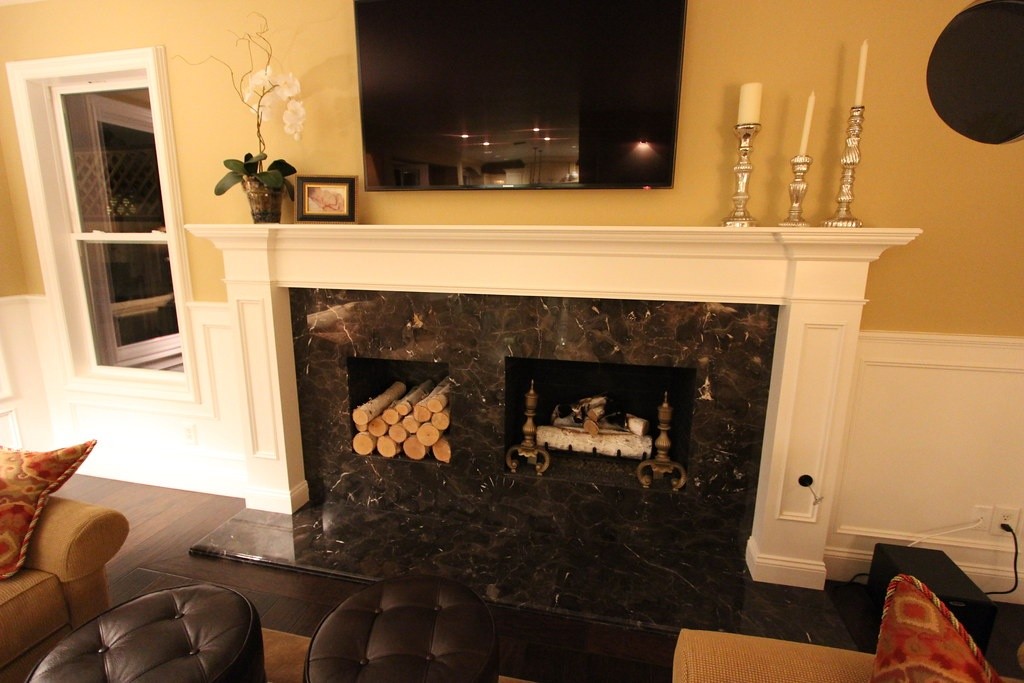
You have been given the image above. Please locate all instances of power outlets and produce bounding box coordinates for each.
[989,505,1019,537]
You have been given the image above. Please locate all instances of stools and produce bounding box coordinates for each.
[300,570,505,683]
[23,575,267,683]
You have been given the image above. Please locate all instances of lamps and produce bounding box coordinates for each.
[632,114,657,142]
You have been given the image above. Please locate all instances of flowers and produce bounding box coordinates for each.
[168,11,308,201]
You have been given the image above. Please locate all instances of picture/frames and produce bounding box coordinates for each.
[295,173,359,225]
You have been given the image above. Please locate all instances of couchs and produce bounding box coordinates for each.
[0,496,136,683]
[672,628,1024,683]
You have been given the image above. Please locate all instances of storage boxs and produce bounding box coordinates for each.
[869,543,999,659]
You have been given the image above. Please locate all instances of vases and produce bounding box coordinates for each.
[242,173,285,221]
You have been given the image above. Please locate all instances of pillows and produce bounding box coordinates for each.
[868,575,1004,683]
[0,438,98,580]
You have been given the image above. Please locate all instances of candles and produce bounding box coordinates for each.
[794,89,818,157]
[732,81,767,126]
[848,39,871,108]
